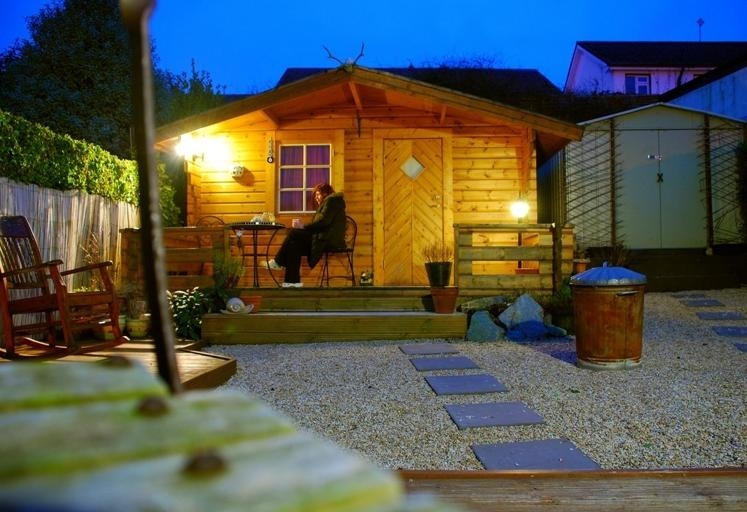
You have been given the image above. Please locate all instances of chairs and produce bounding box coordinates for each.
[1,215,131,362]
[197,216,244,289]
[320,215,357,287]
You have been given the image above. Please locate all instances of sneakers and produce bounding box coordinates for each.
[257,258,284,272]
[278,281,303,288]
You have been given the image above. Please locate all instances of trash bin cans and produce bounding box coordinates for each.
[569,262,647,371]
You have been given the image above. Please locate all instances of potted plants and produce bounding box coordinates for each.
[423,239,455,286]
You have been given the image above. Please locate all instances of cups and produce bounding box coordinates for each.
[292,218,302,229]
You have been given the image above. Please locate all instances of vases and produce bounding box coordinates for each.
[573,259,590,273]
[431,287,458,313]
[240,295,262,313]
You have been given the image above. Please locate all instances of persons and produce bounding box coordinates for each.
[258,182,348,289]
